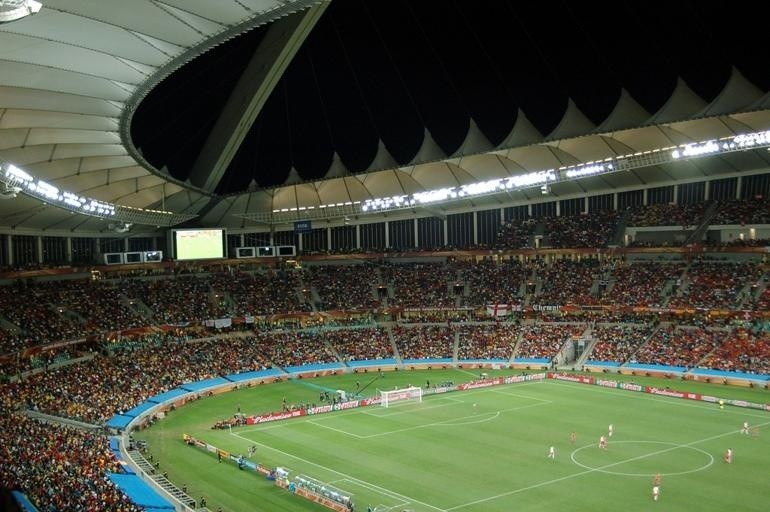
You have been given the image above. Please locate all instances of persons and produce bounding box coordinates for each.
[1,255,339,511]
[571,432,576,442]
[653,472,661,501]
[725,448,731,464]
[609,424,613,438]
[496,197,770,247]
[548,446,555,460]
[319,242,770,407]
[719,399,724,409]
[600,434,605,448]
[741,422,748,435]
